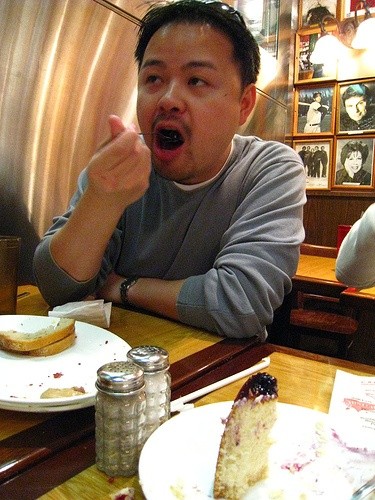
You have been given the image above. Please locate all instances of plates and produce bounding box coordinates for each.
[0,314,133,413]
[137,400,375,500]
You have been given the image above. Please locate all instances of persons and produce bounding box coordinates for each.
[335,202,375,288]
[296,85,372,187]
[33,0,307,341]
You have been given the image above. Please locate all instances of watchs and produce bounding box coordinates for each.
[120,275,141,308]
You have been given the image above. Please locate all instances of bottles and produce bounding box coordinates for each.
[93,344,174,479]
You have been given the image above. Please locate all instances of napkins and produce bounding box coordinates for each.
[48,299,113,329]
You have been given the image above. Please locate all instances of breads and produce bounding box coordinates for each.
[213,371,278,500]
[0,317,76,357]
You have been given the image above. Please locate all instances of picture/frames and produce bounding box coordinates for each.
[340,0,375,22]
[293,25,337,84]
[331,135,375,192]
[336,78,375,134]
[298,0,341,30]
[292,138,334,191]
[292,81,338,136]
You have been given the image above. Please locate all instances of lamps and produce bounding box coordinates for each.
[309,0,375,65]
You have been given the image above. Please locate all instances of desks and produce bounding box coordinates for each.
[290,253,349,310]
[0,277,259,487]
[0,342,375,500]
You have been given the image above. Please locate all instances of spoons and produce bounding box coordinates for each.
[136,131,181,143]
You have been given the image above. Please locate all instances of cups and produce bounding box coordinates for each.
[336,224,352,255]
[0,235,22,315]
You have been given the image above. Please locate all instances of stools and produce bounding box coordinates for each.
[279,307,360,361]
